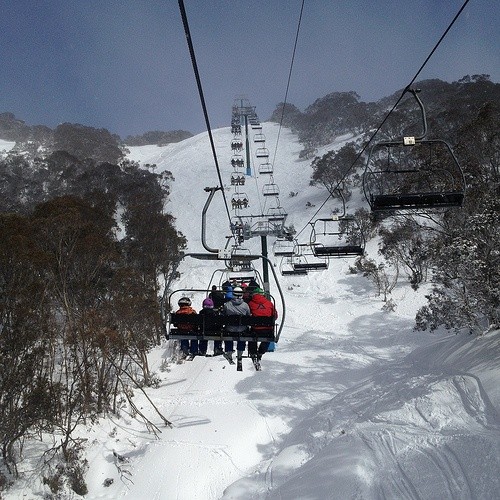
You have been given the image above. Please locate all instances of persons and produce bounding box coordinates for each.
[231,113,241,135]
[231,175,245,185]
[231,198,248,209]
[196,298,225,357]
[173,297,198,356]
[221,287,250,371]
[230,221,249,233]
[207,279,261,309]
[247,284,278,371]
[231,141,243,150]
[231,159,244,167]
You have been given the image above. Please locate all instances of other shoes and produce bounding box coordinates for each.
[255,363,262,371]
[214,348,224,355]
[252,354,258,365]
[238,350,243,357]
[184,350,189,355]
[190,352,197,355]
[199,350,206,355]
[227,352,232,360]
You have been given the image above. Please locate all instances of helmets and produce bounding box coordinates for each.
[252,288,263,295]
[178,297,191,307]
[202,298,214,309]
[233,287,243,299]
